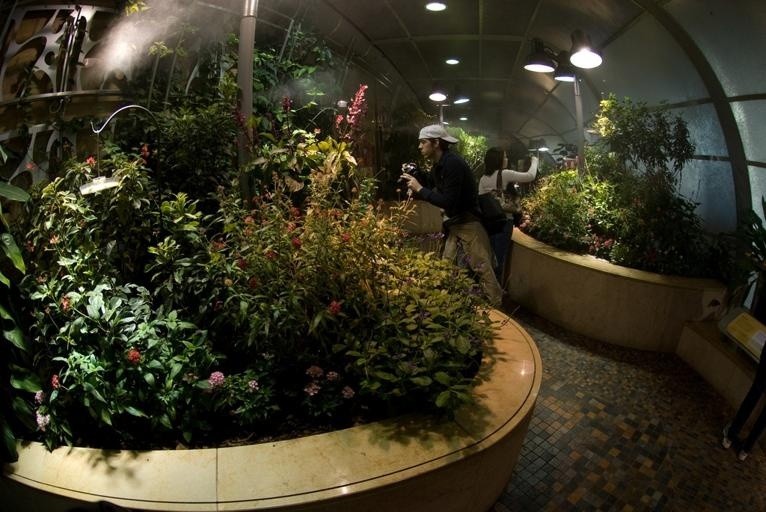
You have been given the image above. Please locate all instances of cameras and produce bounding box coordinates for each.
[397,162,419,188]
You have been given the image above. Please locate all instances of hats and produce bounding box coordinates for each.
[417,124,460,145]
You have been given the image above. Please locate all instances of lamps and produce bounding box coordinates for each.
[520,25,603,83]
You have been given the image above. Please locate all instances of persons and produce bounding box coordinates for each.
[401,124,502,311]
[477,147,539,275]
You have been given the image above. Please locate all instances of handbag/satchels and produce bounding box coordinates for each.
[476,168,523,226]
